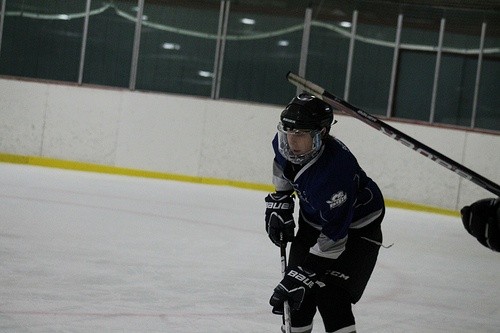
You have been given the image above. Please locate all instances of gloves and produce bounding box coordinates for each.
[270,264,327,314]
[264,192,295,246]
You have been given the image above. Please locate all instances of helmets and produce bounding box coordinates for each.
[280,92,333,143]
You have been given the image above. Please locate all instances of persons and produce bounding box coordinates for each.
[264,92,386,333]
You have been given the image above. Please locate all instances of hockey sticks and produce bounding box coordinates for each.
[280,233,292,333]
[287,71,500,197]
